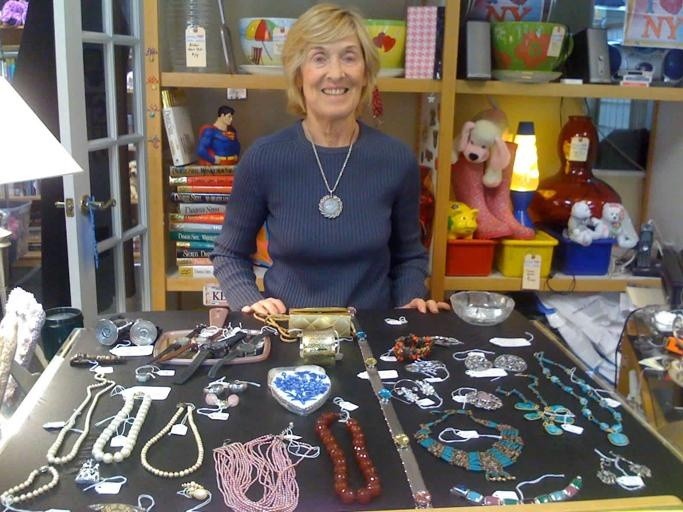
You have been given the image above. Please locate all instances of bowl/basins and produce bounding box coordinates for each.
[363,19,407,66]
[239,19,298,61]
[450,290,516,327]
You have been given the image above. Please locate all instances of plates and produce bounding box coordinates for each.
[240,61,287,78]
[491,66,561,84]
[374,68,405,78]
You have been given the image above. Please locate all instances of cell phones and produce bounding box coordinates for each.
[637,222,655,267]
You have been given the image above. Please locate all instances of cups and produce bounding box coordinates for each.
[493,21,573,69]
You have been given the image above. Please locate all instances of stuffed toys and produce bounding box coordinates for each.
[595,203,639,249]
[450,117,512,189]
[567,201,598,245]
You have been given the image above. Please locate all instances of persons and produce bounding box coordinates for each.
[209,2,451,318]
[196,104,242,165]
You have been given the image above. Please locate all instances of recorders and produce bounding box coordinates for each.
[611,28,683,85]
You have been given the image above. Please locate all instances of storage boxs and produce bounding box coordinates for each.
[550,225,616,275]
[447,239,498,275]
[495,227,559,277]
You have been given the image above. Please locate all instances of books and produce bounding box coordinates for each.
[162,88,195,166]
[168,165,234,279]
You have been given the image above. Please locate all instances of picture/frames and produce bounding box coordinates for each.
[622,0,682,49]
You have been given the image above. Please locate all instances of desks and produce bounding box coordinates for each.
[8,307,683,509]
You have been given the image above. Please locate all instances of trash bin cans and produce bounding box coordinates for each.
[41,306,84,362]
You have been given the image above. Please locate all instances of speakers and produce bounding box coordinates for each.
[466,23,492,79]
[564,30,611,85]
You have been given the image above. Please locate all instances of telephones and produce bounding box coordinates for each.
[631,224,658,277]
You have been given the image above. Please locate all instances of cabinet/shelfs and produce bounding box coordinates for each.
[436,0,682,306]
[141,0,452,307]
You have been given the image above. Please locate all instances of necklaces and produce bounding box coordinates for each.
[592,445,653,491]
[388,332,528,407]
[413,408,524,481]
[1,465,60,506]
[140,402,205,479]
[211,421,320,512]
[449,475,584,506]
[315,411,380,504]
[200,377,248,411]
[497,372,577,436]
[300,117,358,219]
[45,373,115,465]
[91,391,152,465]
[533,351,629,447]
